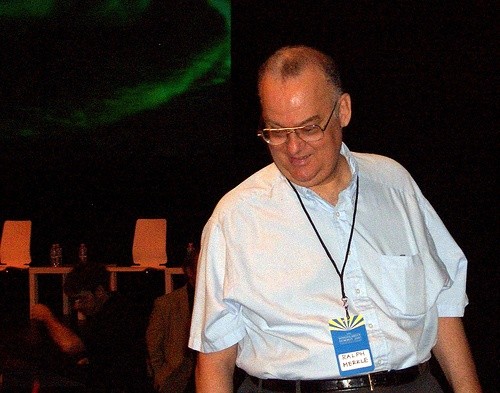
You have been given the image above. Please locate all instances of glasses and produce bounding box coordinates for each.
[257,98,340,144]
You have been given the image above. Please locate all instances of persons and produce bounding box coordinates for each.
[188,46,482,393]
[146,249,200,393]
[31,262,149,393]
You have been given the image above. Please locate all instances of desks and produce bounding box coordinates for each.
[29,267,185,320]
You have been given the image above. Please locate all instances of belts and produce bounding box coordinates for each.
[249,365,422,393]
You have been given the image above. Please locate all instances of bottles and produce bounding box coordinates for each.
[78,243,87,262]
[186,242,195,256]
[50,244,62,268]
